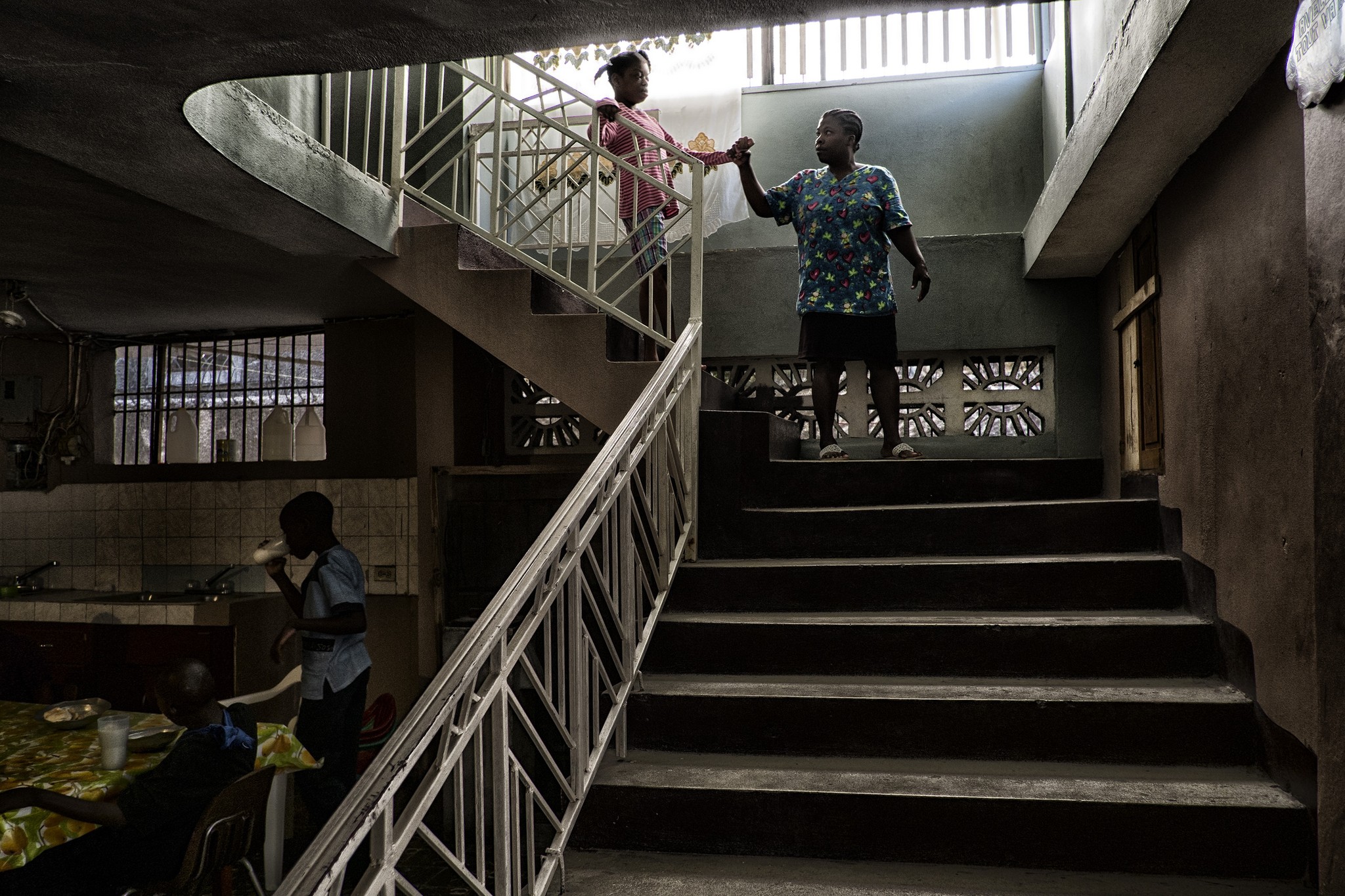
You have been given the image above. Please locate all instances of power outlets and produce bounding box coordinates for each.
[375,567,395,581]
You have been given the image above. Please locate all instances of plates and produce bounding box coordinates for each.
[33,697,111,728]
[127,725,185,751]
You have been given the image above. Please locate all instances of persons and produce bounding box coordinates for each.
[255,491,373,869]
[0,662,256,896]
[588,48,756,367]
[737,107,933,460]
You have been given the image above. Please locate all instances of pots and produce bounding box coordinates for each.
[0,555,60,599]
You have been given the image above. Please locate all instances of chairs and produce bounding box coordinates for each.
[124,763,267,896]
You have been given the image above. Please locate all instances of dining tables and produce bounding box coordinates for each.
[0,699,189,874]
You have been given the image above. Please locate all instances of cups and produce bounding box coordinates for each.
[97,714,128,771]
[252,534,291,565]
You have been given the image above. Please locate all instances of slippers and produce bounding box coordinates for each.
[884,443,926,460]
[818,444,849,460]
